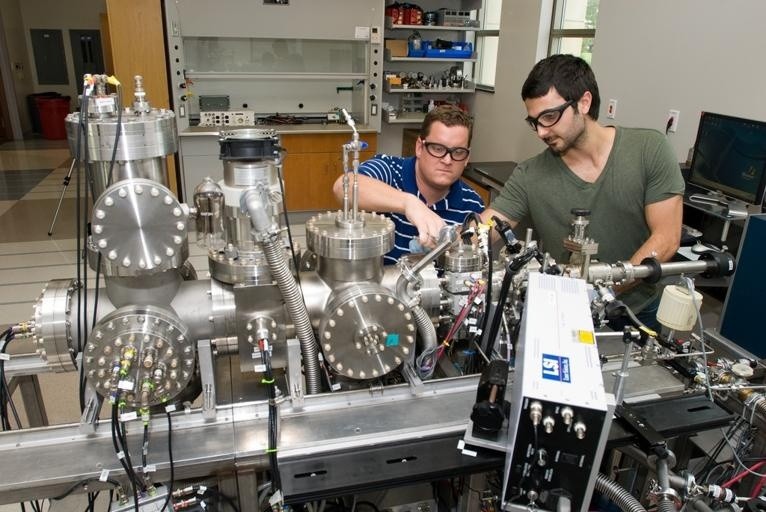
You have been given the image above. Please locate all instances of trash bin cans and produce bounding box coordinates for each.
[27,92,71,140]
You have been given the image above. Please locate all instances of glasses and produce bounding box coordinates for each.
[421,140,471,162]
[525,100,574,131]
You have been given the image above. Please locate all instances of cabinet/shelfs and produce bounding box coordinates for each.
[281,133,377,212]
[384,15,480,125]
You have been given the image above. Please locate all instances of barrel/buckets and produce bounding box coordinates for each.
[34,95,71,139]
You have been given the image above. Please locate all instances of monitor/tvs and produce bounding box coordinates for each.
[688,111,766,217]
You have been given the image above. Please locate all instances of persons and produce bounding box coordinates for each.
[455,55,686,347]
[333,105,486,271]
